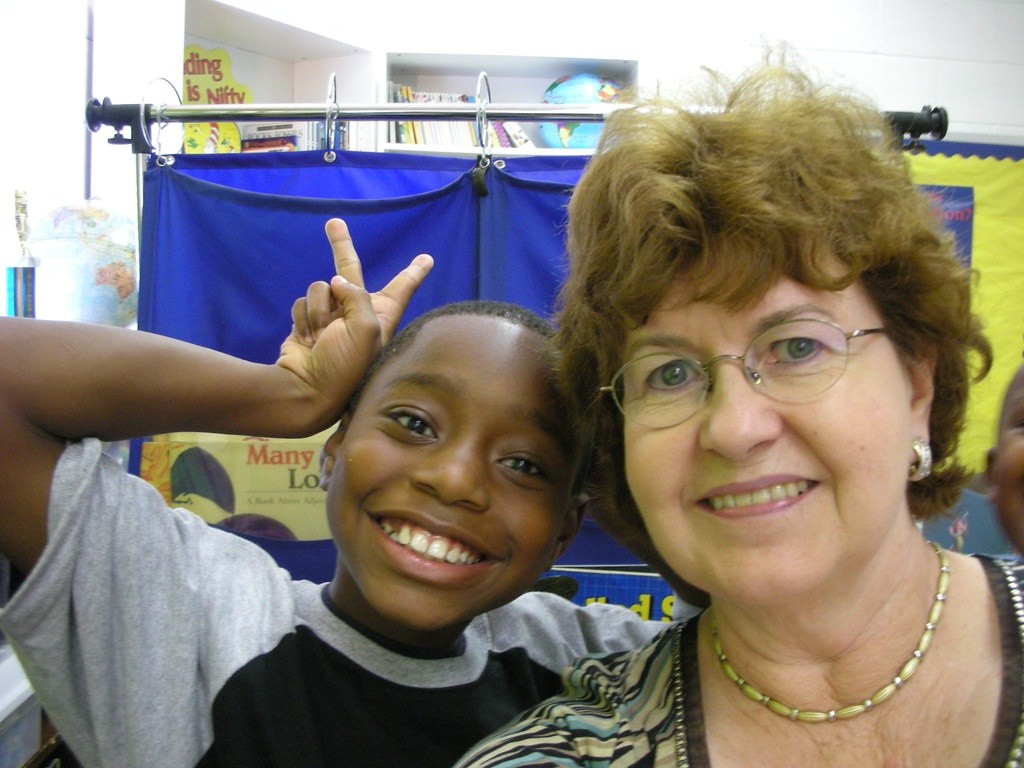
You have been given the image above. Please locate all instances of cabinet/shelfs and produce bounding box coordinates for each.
[0,0,646,218]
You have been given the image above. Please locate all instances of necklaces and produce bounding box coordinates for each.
[710,538,951,723]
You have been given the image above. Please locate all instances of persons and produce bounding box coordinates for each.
[0,218,679,768]
[454,44,1024,768]
[986,366,1024,555]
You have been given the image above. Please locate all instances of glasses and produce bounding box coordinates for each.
[600,318,895,430]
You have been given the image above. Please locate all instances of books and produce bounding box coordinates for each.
[239,80,535,153]
[4,267,36,319]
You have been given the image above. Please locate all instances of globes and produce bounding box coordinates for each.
[540,74,622,149]
